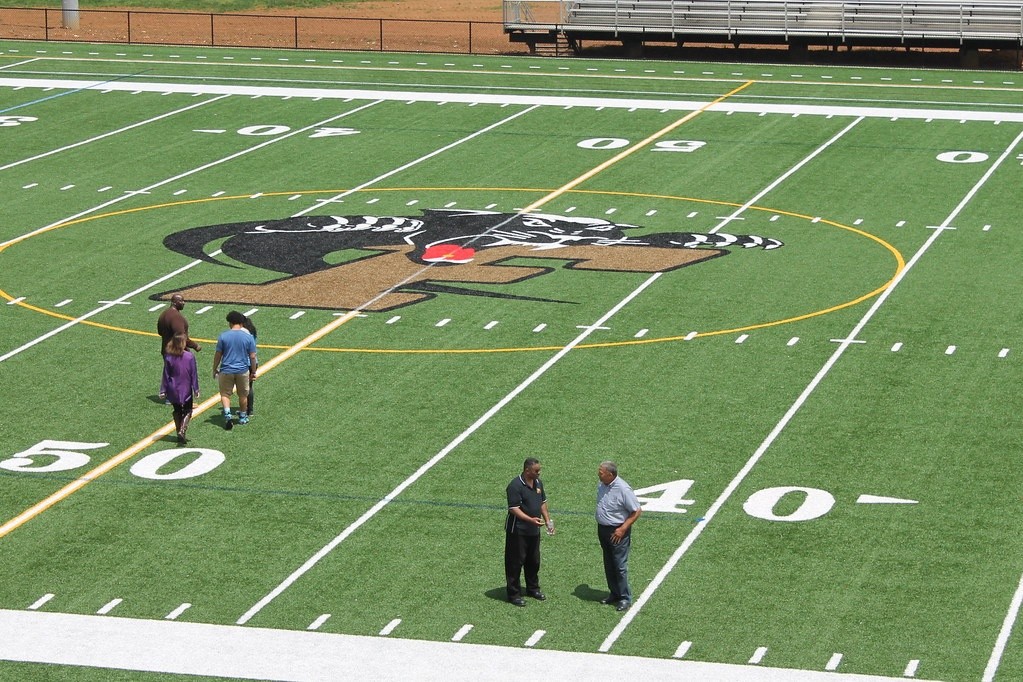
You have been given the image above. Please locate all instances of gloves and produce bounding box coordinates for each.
[159,393,167,400]
[195,392,200,399]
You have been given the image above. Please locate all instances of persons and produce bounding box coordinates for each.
[595,460,640,610]
[504,458,555,606]
[156,295,259,444]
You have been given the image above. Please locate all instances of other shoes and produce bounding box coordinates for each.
[239,411,250,423]
[165,398,172,405]
[225,412,233,428]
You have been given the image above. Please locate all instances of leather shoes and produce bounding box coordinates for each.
[617,599,632,611]
[527,591,546,600]
[509,598,526,606]
[600,596,620,604]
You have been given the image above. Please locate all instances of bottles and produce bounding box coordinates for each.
[215,368,220,378]
[548,520,555,535]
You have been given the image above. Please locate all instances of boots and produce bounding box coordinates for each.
[177,412,190,443]
[173,411,183,442]
